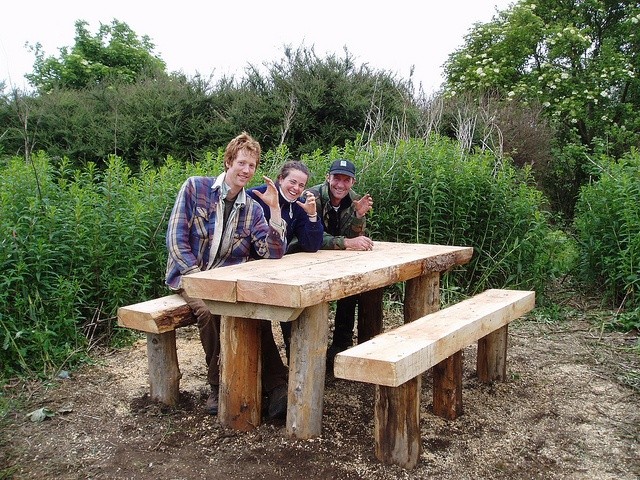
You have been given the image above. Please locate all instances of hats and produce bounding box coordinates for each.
[328,160,357,181]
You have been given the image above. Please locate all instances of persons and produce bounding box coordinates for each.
[302,160,374,368]
[164,129,287,414]
[244,160,323,366]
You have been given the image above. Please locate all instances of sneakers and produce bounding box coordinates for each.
[206,385,220,414]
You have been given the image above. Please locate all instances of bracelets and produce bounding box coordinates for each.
[307,212,319,217]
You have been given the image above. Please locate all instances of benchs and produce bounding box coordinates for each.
[333,287,537,470]
[116,291,198,409]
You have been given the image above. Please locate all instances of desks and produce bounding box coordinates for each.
[182,240,474,441]
